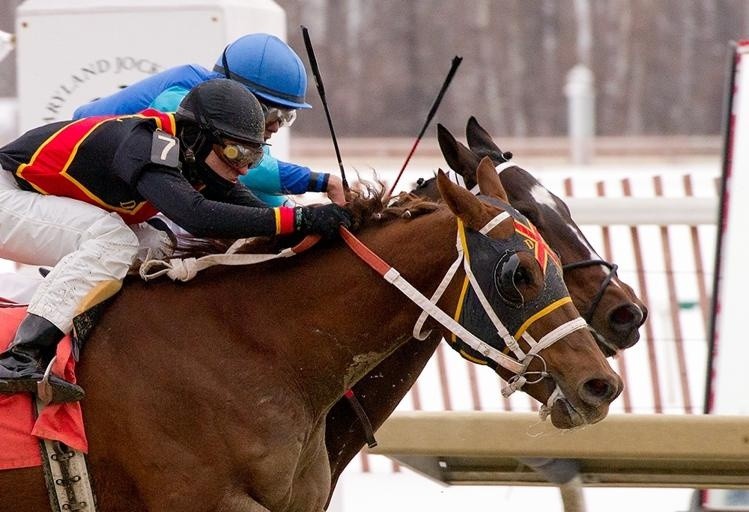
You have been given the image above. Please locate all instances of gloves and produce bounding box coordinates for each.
[294,199,353,237]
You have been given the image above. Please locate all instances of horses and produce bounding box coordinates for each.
[325,115,653,512]
[2,124,623,512]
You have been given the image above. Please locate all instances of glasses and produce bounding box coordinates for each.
[213,138,265,172]
[259,101,298,130]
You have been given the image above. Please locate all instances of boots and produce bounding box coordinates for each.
[0,311,87,405]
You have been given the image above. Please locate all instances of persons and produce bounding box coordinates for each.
[0,78,361,405]
[72,30,359,259]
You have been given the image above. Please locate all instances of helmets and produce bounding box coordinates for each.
[174,76,273,150]
[210,30,315,112]
